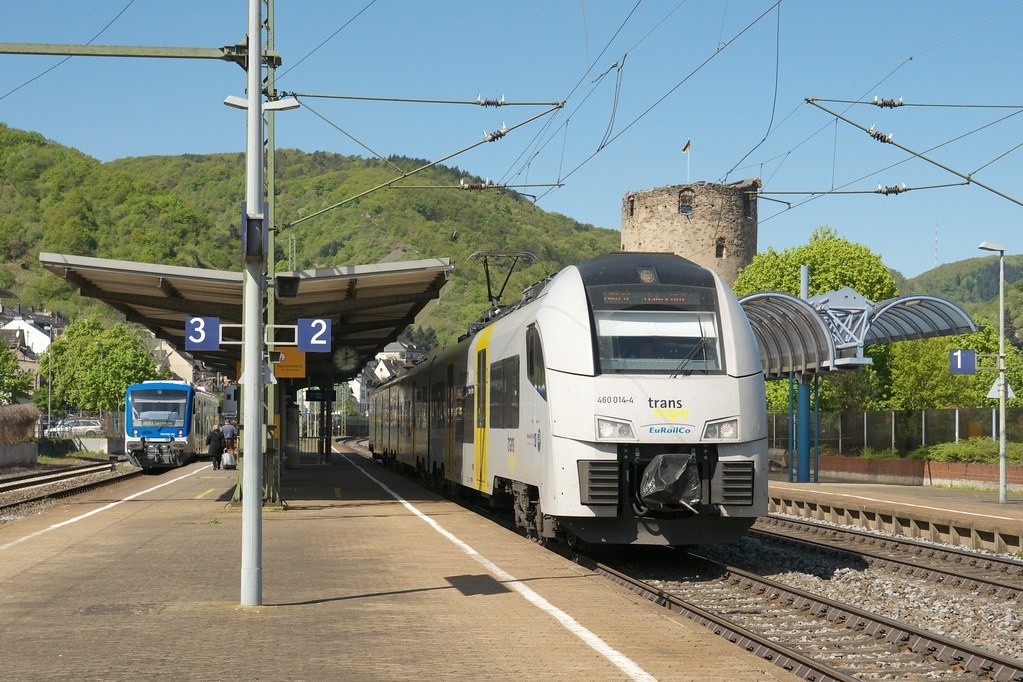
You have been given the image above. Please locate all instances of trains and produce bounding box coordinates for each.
[369,251,769,546]
[125,380,220,469]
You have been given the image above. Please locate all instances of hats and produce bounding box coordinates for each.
[213,425,219,431]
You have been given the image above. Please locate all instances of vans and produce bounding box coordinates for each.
[44,419,104,438]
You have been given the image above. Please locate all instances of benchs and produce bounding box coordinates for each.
[768,448,787,475]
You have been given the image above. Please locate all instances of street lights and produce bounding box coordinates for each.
[223,96,301,606]
[44,325,52,438]
[977,242,1008,504]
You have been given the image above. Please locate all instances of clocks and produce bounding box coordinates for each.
[332,345,361,375]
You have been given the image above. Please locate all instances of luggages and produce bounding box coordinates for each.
[222,449,237,470]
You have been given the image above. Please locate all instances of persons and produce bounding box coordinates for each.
[206,424,227,470]
[220,418,238,454]
[636,338,655,358]
